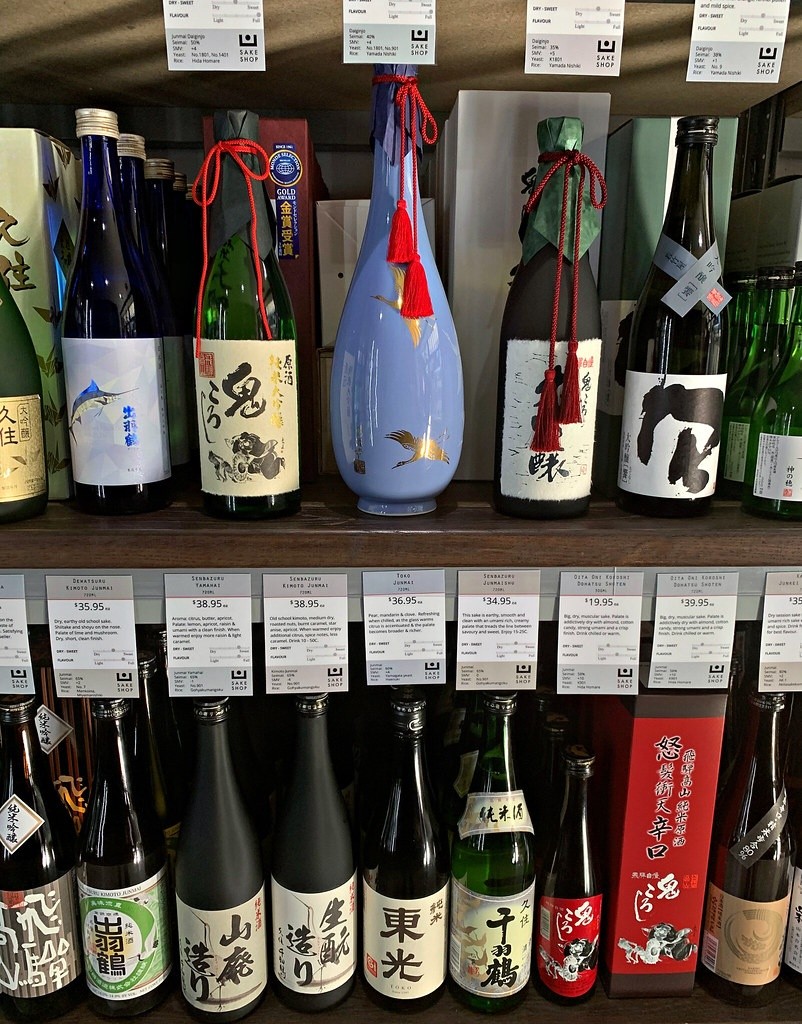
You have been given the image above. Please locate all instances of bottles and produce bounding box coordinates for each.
[2,694,800,1023]
[0,67,802,521]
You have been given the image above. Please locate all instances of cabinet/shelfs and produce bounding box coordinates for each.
[0,0,802,1024]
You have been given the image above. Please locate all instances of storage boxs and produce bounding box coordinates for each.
[588,661,728,997]
[203,90,802,503]
[0,128,86,501]
